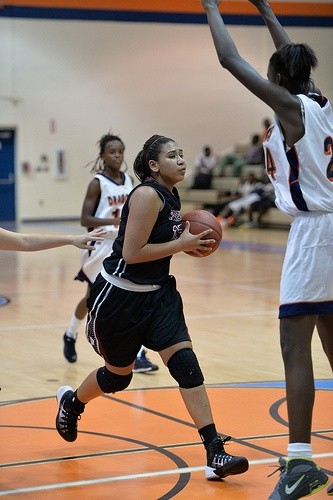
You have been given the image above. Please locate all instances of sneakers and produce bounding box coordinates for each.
[64,334,77,362]
[55,385,81,442]
[204,436,249,479]
[267,456,329,500]
[132,350,159,373]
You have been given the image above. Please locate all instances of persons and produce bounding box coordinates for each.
[64,133,159,373]
[0,226,107,252]
[56,135,249,481]
[200,0,333,500]
[190,119,278,228]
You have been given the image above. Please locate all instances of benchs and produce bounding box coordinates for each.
[173,141,293,226]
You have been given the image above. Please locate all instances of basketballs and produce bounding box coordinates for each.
[180,208,222,258]
